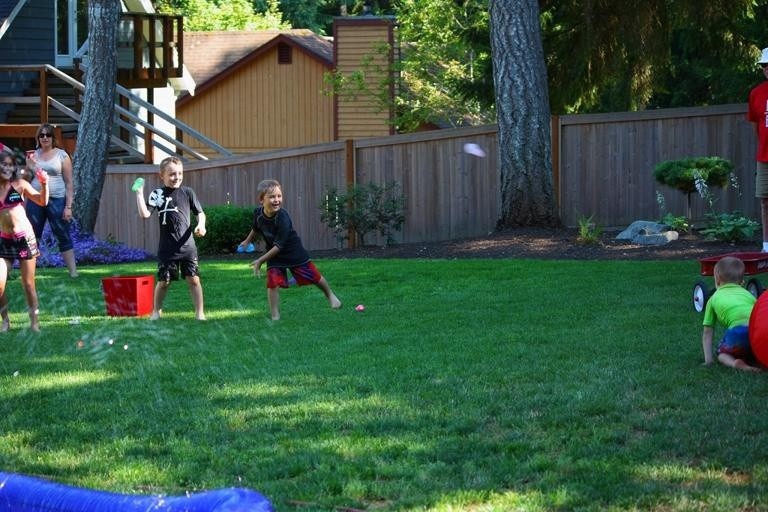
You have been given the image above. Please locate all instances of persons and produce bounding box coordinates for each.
[239,179,342,324]
[702,257,762,373]
[21,123,79,278]
[747,48,768,254]
[0,151,50,333]
[135,157,208,322]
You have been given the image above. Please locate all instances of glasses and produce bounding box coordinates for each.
[37,133,51,139]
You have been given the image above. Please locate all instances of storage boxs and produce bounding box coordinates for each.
[101,275,154,317]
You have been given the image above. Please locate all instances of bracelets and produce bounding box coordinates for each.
[64,205,72,209]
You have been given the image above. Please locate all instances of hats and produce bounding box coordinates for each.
[755,46,767,68]
